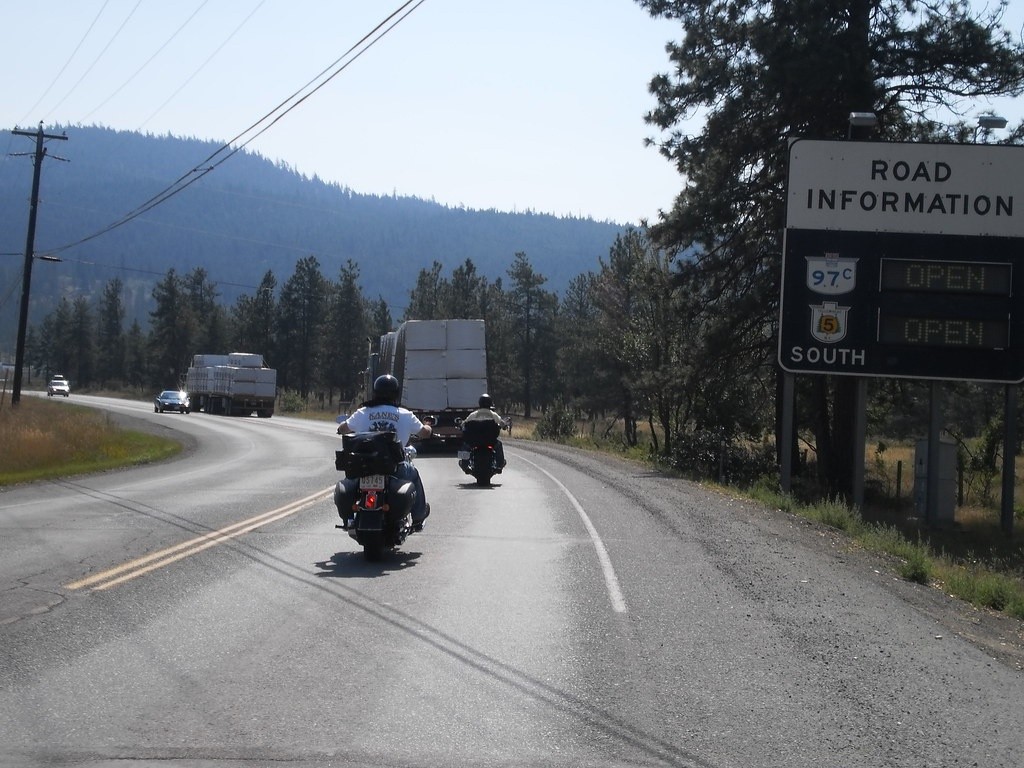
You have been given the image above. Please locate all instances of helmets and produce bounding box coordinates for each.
[479,394,492,408]
[374,375,399,400]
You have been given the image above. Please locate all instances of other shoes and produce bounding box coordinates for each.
[497,460,506,470]
[413,503,430,524]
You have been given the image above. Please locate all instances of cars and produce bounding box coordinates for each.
[155,390,190,414]
[48,380,70,397]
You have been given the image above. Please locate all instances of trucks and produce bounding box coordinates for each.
[179,353,276,418]
[350,320,487,447]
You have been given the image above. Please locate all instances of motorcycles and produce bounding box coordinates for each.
[334,415,444,560]
[454,416,511,485]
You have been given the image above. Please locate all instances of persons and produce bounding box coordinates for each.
[338,375,432,525]
[460,394,509,469]
